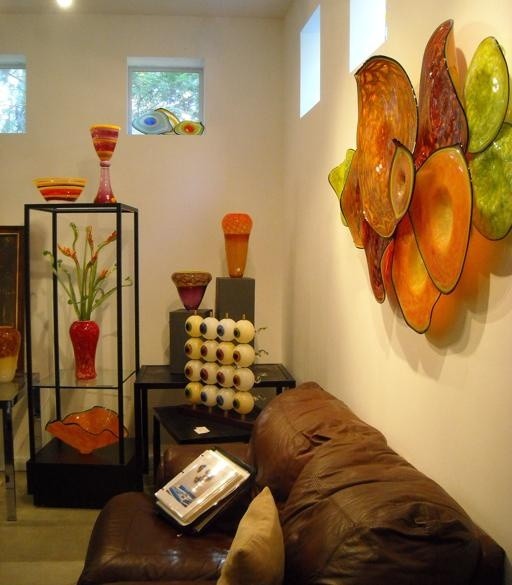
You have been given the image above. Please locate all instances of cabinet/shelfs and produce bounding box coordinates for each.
[25,204,138,509]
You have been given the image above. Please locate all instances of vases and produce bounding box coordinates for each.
[171,273,211,309]
[1,325,21,383]
[223,213,252,278]
[70,322,99,379]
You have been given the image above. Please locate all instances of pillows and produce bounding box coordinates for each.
[214,486,286,584]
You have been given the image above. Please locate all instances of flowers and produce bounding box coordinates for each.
[43,222,133,321]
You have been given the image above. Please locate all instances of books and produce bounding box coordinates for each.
[153,446,257,538]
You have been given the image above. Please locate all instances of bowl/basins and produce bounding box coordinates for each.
[34,177,87,204]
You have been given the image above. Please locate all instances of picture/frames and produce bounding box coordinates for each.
[1,226,24,377]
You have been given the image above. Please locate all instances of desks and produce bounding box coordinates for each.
[1,372,42,520]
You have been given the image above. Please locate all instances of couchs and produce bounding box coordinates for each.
[77,382,506,584]
